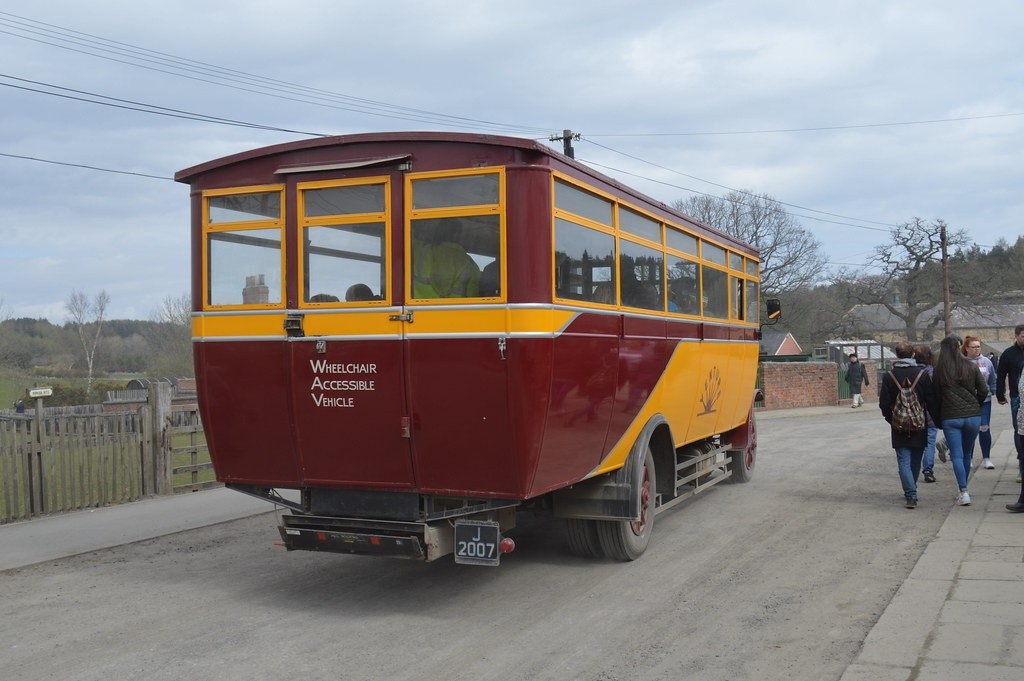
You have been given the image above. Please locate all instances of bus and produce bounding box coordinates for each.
[173,131,784,567]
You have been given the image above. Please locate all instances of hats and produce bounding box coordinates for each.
[850,354,857,357]
[346,284,374,300]
[990,352,994,355]
[948,333,963,345]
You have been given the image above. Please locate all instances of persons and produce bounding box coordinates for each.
[933,338,989,505]
[961,335,996,469]
[912,345,939,483]
[1005,362,1024,513]
[988,352,997,375]
[844,353,869,408]
[309,218,716,319]
[996,325,1024,484]
[934,334,964,463]
[879,342,943,509]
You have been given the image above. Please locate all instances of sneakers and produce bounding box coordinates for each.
[956,491,971,505]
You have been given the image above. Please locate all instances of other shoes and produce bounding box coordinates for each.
[936,441,948,462]
[852,405,855,408]
[1006,502,1024,512]
[925,471,936,482]
[858,401,864,407]
[904,497,918,507]
[983,459,995,469]
[1015,473,1022,483]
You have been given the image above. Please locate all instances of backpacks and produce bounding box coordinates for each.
[887,369,927,438]
